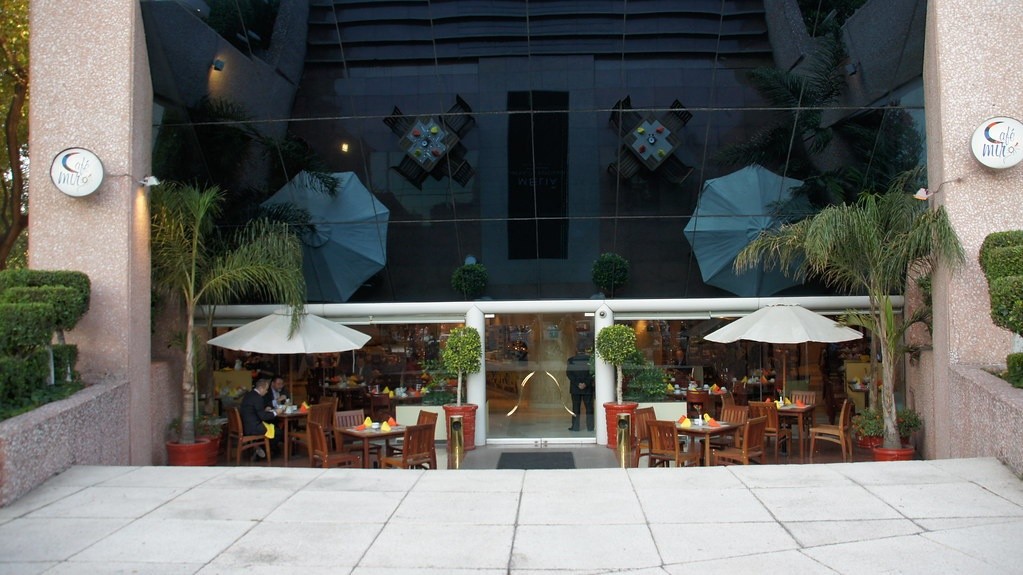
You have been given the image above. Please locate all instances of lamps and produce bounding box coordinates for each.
[139,174,160,188]
[913,187,928,201]
[212,59,225,72]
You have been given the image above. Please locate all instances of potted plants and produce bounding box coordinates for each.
[451,261,490,298]
[715,19,850,181]
[729,192,966,460]
[590,250,634,298]
[148,178,310,467]
[442,325,483,449]
[161,96,315,171]
[595,325,640,450]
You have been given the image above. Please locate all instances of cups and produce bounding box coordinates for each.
[401,392,407,397]
[703,384,708,388]
[372,423,379,428]
[721,387,727,392]
[278,399,297,413]
[694,419,699,425]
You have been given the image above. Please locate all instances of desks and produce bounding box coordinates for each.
[779,402,817,460]
[397,113,460,174]
[333,422,404,468]
[671,420,742,466]
[274,410,307,463]
[622,113,683,171]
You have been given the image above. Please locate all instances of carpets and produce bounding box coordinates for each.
[494,451,577,469]
[505,88,570,260]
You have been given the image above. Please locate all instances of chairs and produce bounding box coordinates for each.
[439,154,466,177]
[383,106,412,136]
[444,94,474,140]
[634,390,854,466]
[609,94,643,132]
[658,154,696,185]
[225,393,438,471]
[389,155,424,184]
[662,98,693,131]
[607,155,643,186]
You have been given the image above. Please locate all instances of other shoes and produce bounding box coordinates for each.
[568,427,579,431]
[255,447,265,458]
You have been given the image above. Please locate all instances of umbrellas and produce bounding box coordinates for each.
[252,170,390,301]
[683,164,822,298]
[205,304,373,407]
[704,305,864,428]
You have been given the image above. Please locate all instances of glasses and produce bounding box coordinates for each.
[275,382,284,386]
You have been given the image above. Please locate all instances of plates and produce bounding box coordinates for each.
[696,388,702,390]
[680,388,688,390]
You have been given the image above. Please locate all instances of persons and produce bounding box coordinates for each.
[314,350,381,411]
[239,373,298,458]
[361,345,394,384]
[733,343,752,378]
[566,342,594,432]
[750,345,764,369]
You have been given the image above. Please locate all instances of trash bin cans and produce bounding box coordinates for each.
[686,390,710,418]
[371,393,396,423]
